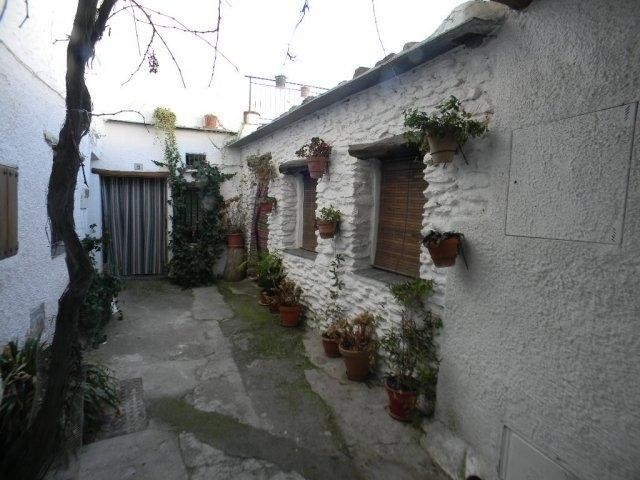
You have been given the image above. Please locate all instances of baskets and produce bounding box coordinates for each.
[318,221,337,239]
[428,237,458,267]
[307,156,325,178]
[261,203,272,213]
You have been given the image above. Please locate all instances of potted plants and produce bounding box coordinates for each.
[246,96,491,185]
[250,193,470,271]
[233,247,443,423]
